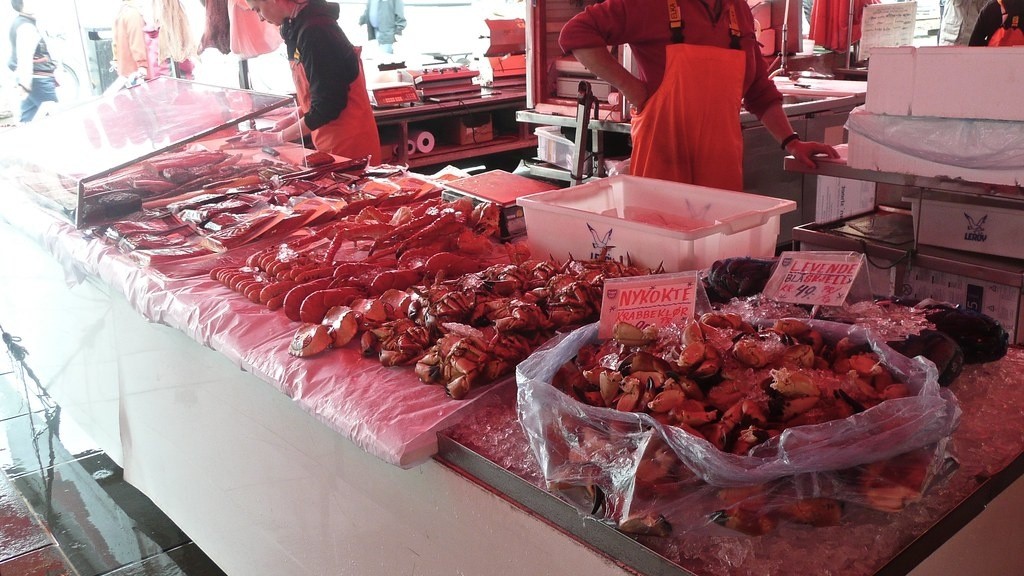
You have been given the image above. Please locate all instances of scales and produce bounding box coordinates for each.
[441,169,562,244]
[366,81,421,108]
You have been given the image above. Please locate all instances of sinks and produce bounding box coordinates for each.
[735,94,827,112]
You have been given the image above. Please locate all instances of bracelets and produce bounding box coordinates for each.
[781,132,801,150]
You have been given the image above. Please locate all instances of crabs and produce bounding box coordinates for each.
[361,246,668,400]
[546,316,962,537]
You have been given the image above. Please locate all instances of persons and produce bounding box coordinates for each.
[558,0,841,193]
[969,0,1024,46]
[240,0,381,167]
[358,0,407,54]
[7,0,60,122]
[114,0,149,77]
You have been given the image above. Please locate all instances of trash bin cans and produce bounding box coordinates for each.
[84,27,118,95]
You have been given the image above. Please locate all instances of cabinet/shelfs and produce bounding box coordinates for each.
[786,146,1024,349]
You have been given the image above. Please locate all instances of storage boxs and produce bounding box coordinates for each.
[449,113,493,145]
[846,43,1024,190]
[800,240,1020,346]
[902,189,1024,260]
[747,0,776,56]
[533,124,575,167]
[516,174,796,275]
